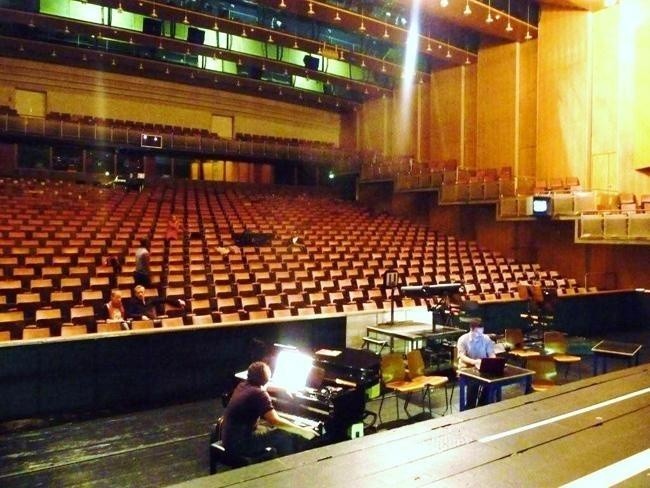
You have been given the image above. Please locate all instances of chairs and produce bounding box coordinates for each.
[379,350,449,421]
[505,329,581,381]
[360,149,649,240]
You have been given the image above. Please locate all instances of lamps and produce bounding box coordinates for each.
[486,0,532,40]
[279,0,472,65]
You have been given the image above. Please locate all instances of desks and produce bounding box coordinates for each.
[456,363,536,411]
[367,321,467,375]
[590,339,642,377]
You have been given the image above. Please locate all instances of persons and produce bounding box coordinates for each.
[457,316,499,410]
[133,234,152,288]
[126,284,185,320]
[100,291,128,323]
[166,214,186,240]
[220,361,322,463]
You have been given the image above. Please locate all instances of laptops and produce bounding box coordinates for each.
[475,358,505,372]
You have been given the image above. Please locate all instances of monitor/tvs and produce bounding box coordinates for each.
[141,134,163,148]
[526,196,552,217]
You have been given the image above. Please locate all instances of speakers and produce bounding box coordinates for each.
[187,28,205,44]
[143,18,161,35]
[304,55,319,70]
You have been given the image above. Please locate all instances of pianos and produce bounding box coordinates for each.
[400,283,462,297]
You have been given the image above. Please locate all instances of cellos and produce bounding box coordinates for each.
[223,342,380,445]
